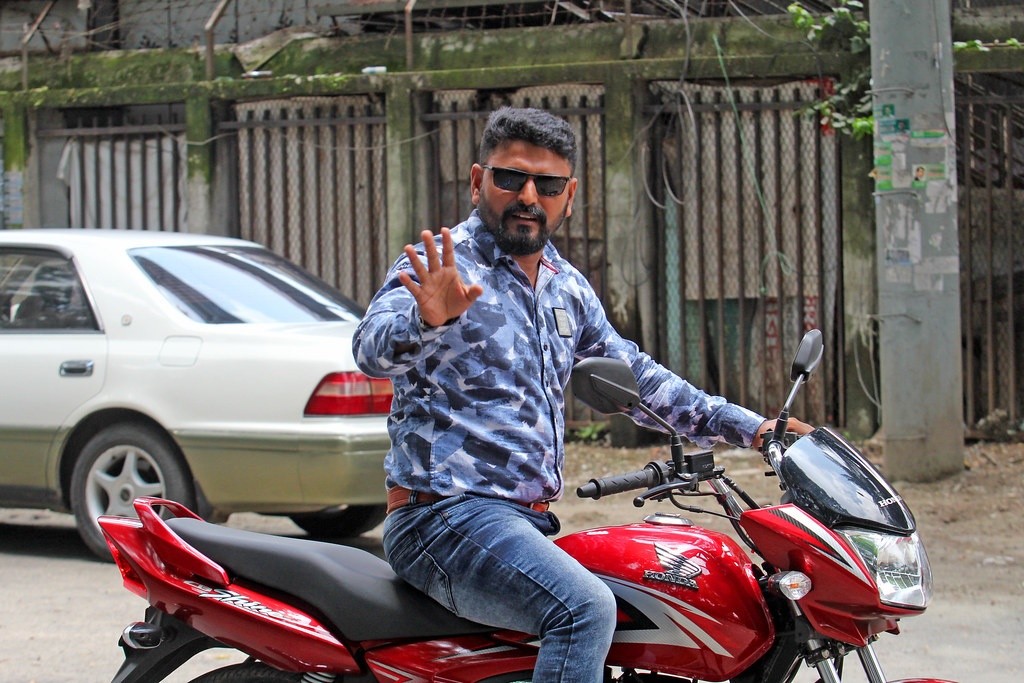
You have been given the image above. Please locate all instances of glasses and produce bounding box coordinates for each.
[482,165,571,198]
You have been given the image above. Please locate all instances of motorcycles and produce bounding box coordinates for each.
[96,327,962,683]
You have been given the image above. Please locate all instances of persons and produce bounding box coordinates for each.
[353,108,817,682]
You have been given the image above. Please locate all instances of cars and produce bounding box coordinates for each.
[0,228,409,568]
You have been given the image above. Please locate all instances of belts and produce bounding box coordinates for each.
[385,485,549,516]
